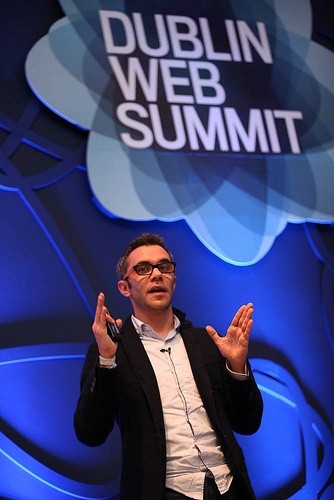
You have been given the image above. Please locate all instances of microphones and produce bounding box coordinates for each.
[160,348,171,355]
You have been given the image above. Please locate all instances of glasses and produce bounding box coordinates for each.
[122,261,176,280]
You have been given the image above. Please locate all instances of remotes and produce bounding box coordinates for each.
[106,321,122,342]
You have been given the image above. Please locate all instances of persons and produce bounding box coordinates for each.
[73,234,264,500]
